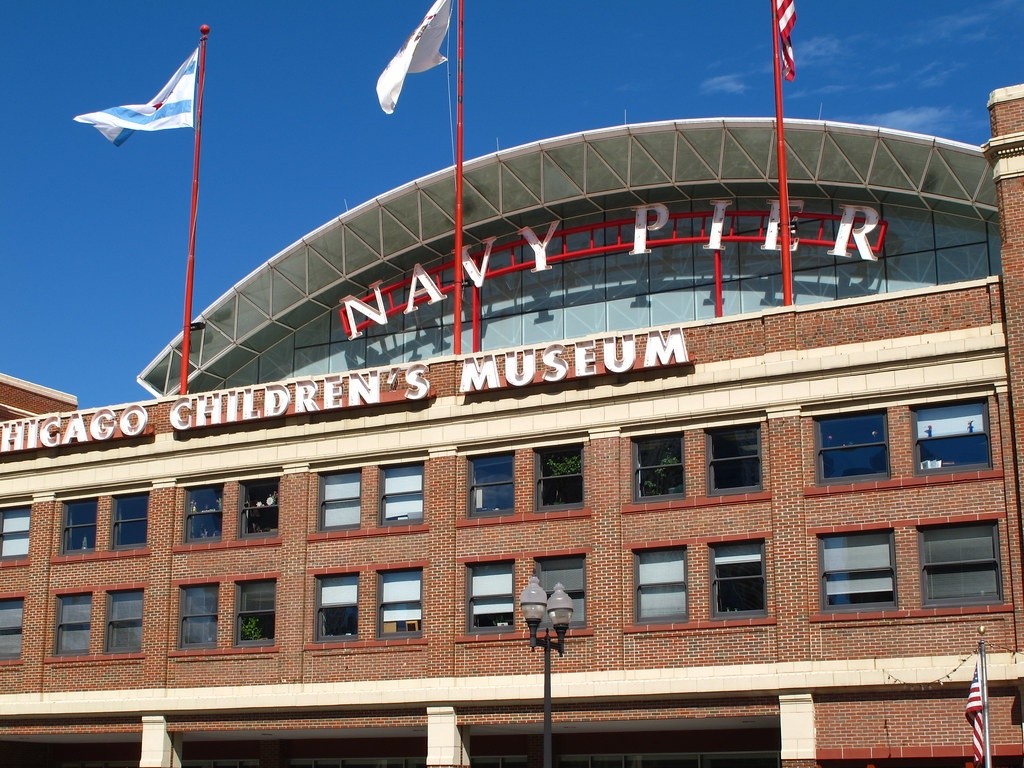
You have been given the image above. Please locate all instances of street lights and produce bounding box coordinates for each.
[518,577,574,768]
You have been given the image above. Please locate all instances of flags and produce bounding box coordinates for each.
[776,0,797,82]
[376,0,453,114]
[964,654,986,768]
[72,47,199,147]
